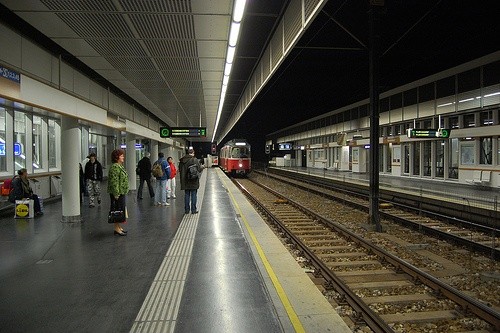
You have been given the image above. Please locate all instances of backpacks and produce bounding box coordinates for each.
[151,160,166,178]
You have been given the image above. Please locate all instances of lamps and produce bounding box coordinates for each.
[211,0,247,144]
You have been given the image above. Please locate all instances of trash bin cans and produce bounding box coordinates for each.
[50,175,63,197]
[29,178,40,195]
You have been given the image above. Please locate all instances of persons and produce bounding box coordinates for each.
[165,157,177,198]
[8,168,44,218]
[179,146,206,215]
[84,153,104,208]
[152,152,171,206]
[135,151,155,200]
[107,148,130,236]
[79,162,85,205]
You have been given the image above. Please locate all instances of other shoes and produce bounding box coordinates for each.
[161,202,171,207]
[136,195,144,200]
[185,209,199,214]
[152,201,162,207]
[167,193,176,199]
[89,200,101,208]
[34,211,43,217]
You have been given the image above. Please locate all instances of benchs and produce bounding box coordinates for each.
[471,170,492,184]
[0,176,17,210]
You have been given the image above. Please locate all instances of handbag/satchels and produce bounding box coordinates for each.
[108,198,126,223]
[187,157,201,180]
[136,163,142,175]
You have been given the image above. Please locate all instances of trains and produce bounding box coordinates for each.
[218,138,251,177]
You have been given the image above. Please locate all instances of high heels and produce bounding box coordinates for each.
[114,228,128,236]
